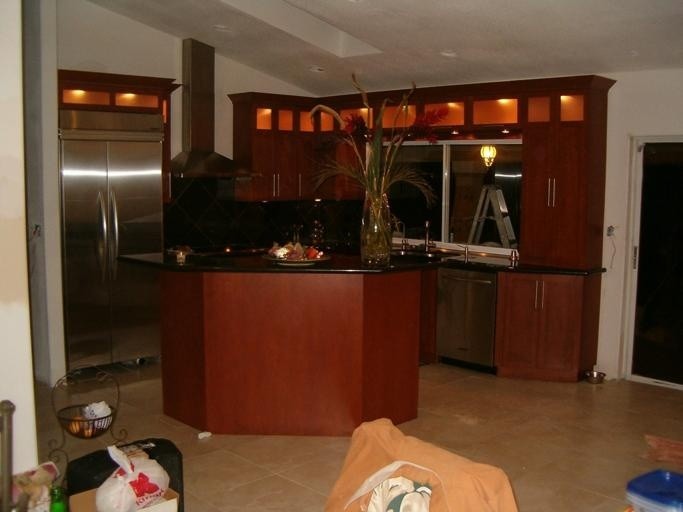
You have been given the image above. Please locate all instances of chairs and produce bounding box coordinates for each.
[324,417,518,512]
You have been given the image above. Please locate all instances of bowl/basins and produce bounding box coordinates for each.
[583,371,606,384]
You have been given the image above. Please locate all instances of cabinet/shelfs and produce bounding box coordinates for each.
[495,266,608,383]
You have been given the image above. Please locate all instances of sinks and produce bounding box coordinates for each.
[390,250,437,258]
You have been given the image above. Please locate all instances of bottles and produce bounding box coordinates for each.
[48,487,66,512]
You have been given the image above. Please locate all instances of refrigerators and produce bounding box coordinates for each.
[57,109,164,373]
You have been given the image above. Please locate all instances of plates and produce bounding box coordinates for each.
[262,254,331,268]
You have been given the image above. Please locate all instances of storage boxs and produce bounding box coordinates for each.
[68,486,180,512]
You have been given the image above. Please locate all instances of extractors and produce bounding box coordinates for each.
[169,37,264,181]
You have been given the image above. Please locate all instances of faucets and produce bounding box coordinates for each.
[424,220,436,252]
[396,221,409,251]
